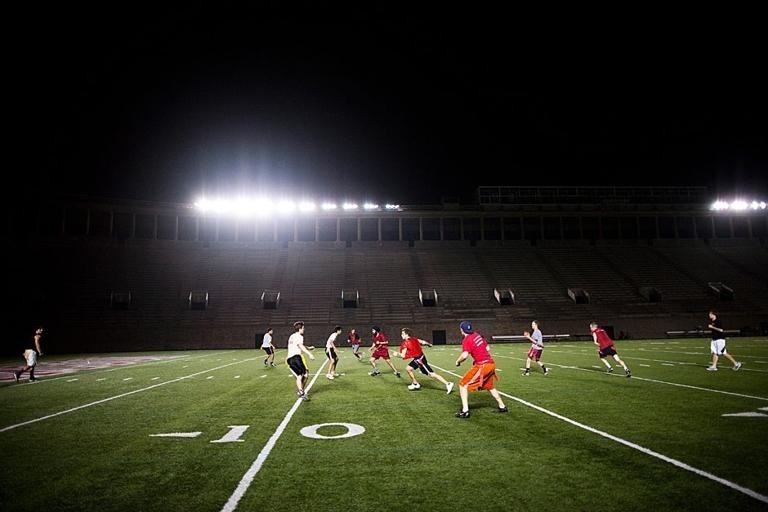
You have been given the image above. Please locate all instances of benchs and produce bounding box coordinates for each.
[3,231,767,351]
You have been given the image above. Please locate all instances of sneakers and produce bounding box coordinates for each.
[543,367,550,376]
[519,371,531,376]
[296,389,312,403]
[368,369,381,377]
[444,381,455,395]
[703,360,743,373]
[606,368,631,378]
[405,381,422,391]
[453,409,471,418]
[326,369,340,381]
[263,359,276,368]
[14,371,40,383]
[494,406,510,412]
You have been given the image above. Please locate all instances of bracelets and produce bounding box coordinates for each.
[38,351,43,353]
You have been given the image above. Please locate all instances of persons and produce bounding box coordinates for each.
[347,327,365,361]
[706,310,742,373]
[324,326,342,380]
[393,328,455,395]
[13,328,44,383]
[368,325,401,379]
[522,320,549,377]
[455,321,509,418]
[286,320,315,402]
[589,321,630,377]
[286,349,311,390]
[259,328,278,366]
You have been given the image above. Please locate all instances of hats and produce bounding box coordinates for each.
[459,321,475,333]
